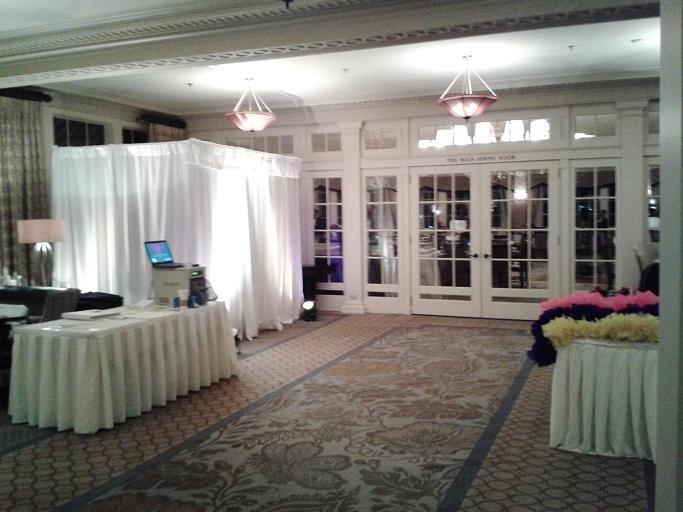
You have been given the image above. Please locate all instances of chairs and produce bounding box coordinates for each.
[509,223,537,287]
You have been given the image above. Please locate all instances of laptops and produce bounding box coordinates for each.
[144,240,184,269]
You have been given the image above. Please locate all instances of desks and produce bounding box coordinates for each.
[546,334,657,464]
[0,284,232,437]
[441,239,508,288]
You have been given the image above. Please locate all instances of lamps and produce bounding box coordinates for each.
[16,218,65,285]
[224,77,275,134]
[437,54,498,126]
